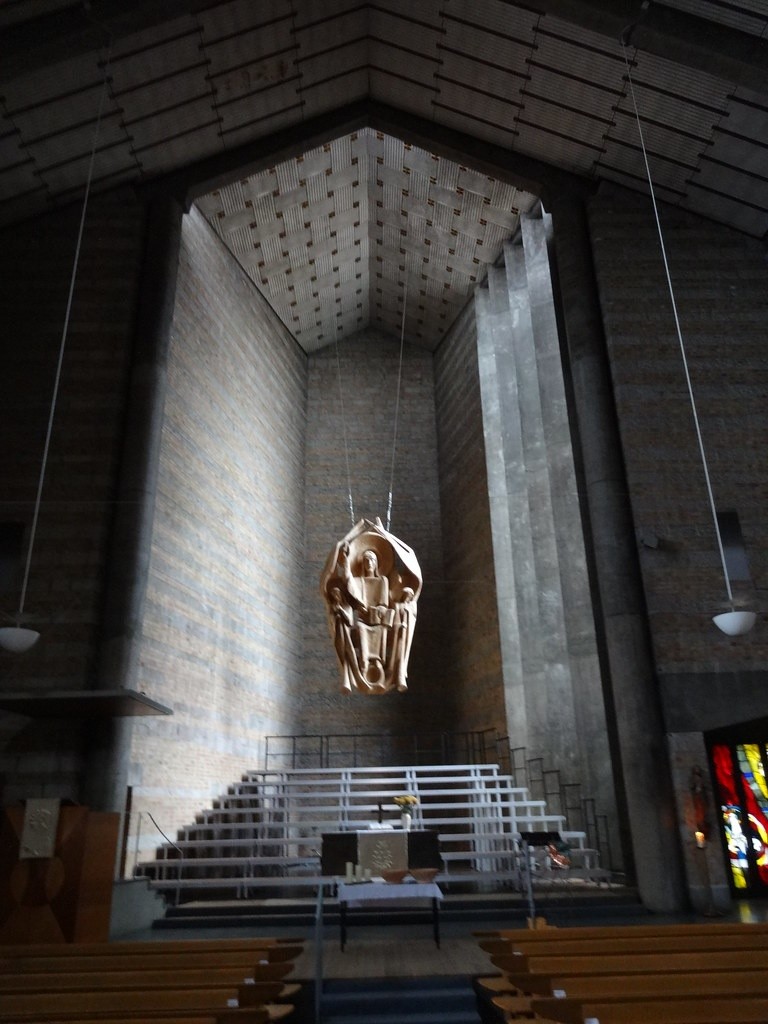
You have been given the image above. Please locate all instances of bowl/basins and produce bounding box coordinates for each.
[409,869,438,883]
[380,869,408,883]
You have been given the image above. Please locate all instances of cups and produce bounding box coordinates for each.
[365,869,372,881]
[346,862,353,883]
[355,865,362,882]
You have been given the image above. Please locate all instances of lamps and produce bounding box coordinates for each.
[0,1,110,654]
[629,0,757,637]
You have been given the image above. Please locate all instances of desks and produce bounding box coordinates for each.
[318,828,444,897]
[335,879,446,954]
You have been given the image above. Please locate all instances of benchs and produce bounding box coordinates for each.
[0,938,307,1023]
[476,924,768,1023]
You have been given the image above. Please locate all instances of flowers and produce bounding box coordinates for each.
[393,795,419,812]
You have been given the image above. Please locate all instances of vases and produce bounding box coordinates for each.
[400,813,411,832]
